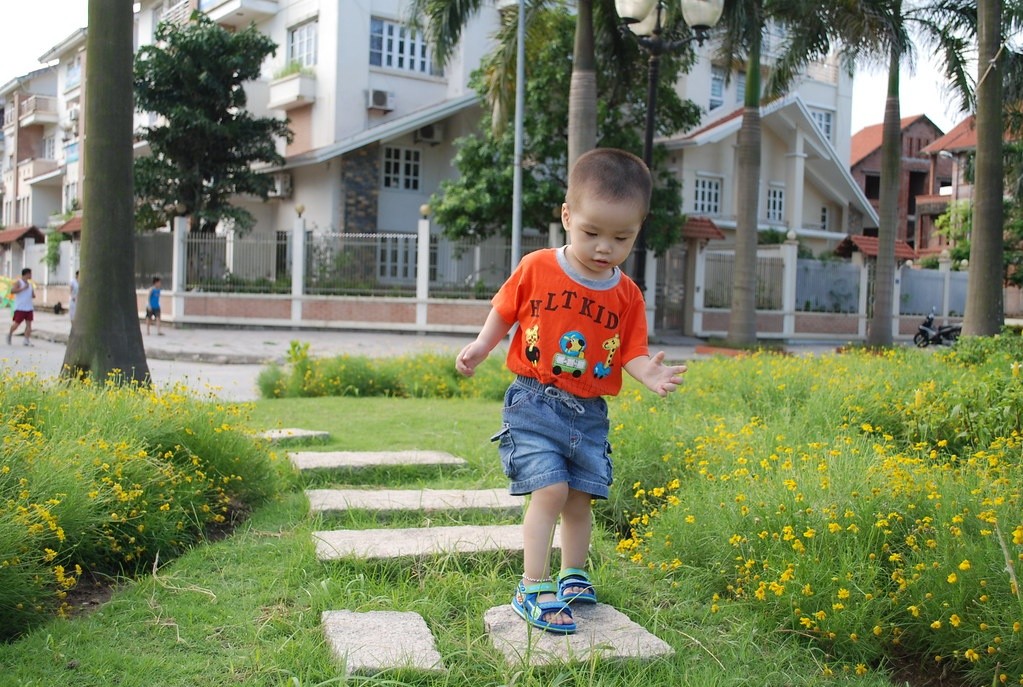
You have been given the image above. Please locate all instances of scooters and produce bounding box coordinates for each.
[914,305,962,349]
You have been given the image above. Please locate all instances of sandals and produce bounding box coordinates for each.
[511,579,577,634]
[556,567,597,605]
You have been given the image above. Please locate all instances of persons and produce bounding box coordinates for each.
[67,271,78,325]
[145,277,166,335]
[6,268,36,346]
[454,147,688,635]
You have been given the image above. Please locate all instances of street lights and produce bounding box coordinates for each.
[615,0,724,307]
[938,149,974,244]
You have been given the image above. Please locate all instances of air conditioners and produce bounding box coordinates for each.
[413,122,444,146]
[266,172,295,199]
[69,108,79,121]
[366,87,396,114]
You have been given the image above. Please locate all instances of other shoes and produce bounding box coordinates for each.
[157,332,165,336]
[146,332,151,336]
[6,334,12,345]
[23,341,34,347]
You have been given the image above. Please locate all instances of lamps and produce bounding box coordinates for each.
[295,203,305,217]
[176,203,187,217]
[419,204,432,219]
[786,230,797,241]
